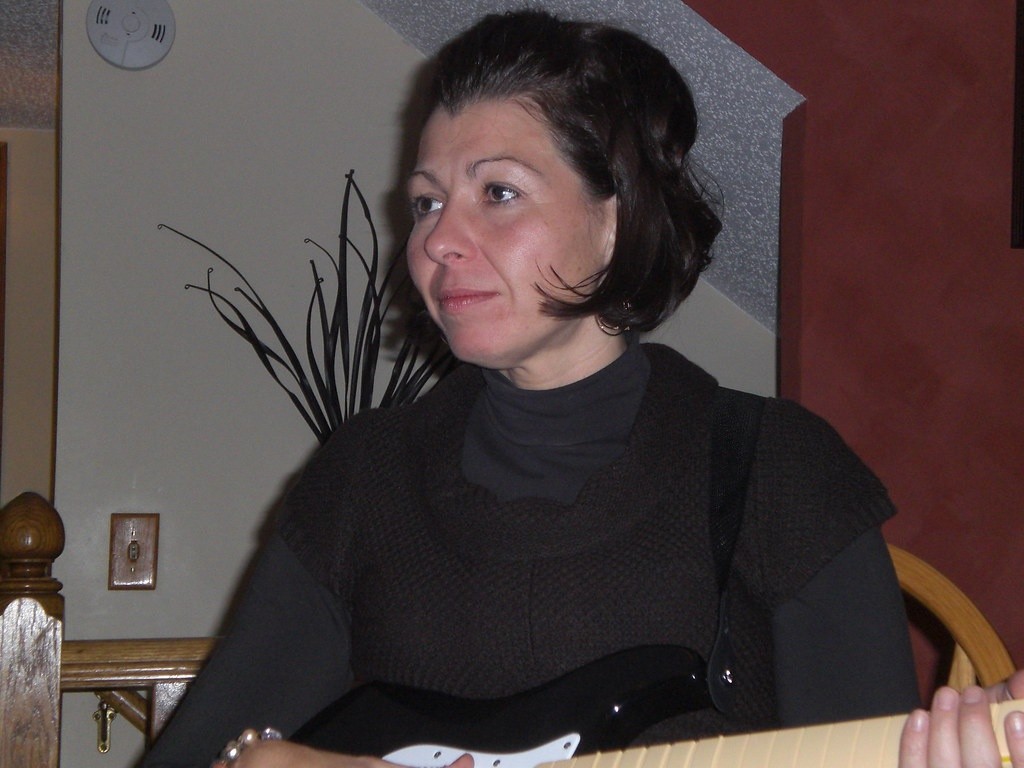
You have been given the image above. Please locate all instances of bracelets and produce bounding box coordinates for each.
[210,725,282,768]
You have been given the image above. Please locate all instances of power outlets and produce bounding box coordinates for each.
[108,512,160,591]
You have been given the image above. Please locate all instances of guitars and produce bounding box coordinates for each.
[282,638,1024,767]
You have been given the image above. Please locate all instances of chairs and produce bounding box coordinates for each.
[886,541,1018,697]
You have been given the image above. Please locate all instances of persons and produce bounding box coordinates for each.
[135,7,1024,768]
